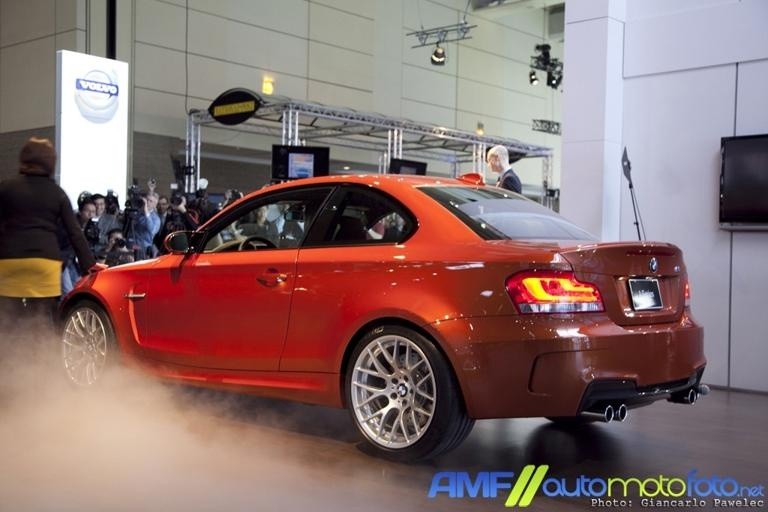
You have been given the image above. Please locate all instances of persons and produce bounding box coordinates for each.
[486,144,523,198]
[56,177,405,311]
[0,136,108,337]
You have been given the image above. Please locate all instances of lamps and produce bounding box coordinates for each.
[405,0,477,66]
[529,8,563,89]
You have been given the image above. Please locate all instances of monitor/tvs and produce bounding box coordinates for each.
[388,158,427,176]
[271,143,330,181]
[719,133,768,231]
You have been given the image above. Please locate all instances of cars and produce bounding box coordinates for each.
[55,171,713,466]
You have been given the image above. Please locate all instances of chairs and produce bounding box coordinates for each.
[332,216,366,241]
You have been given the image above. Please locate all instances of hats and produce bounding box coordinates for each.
[78,192,93,206]
